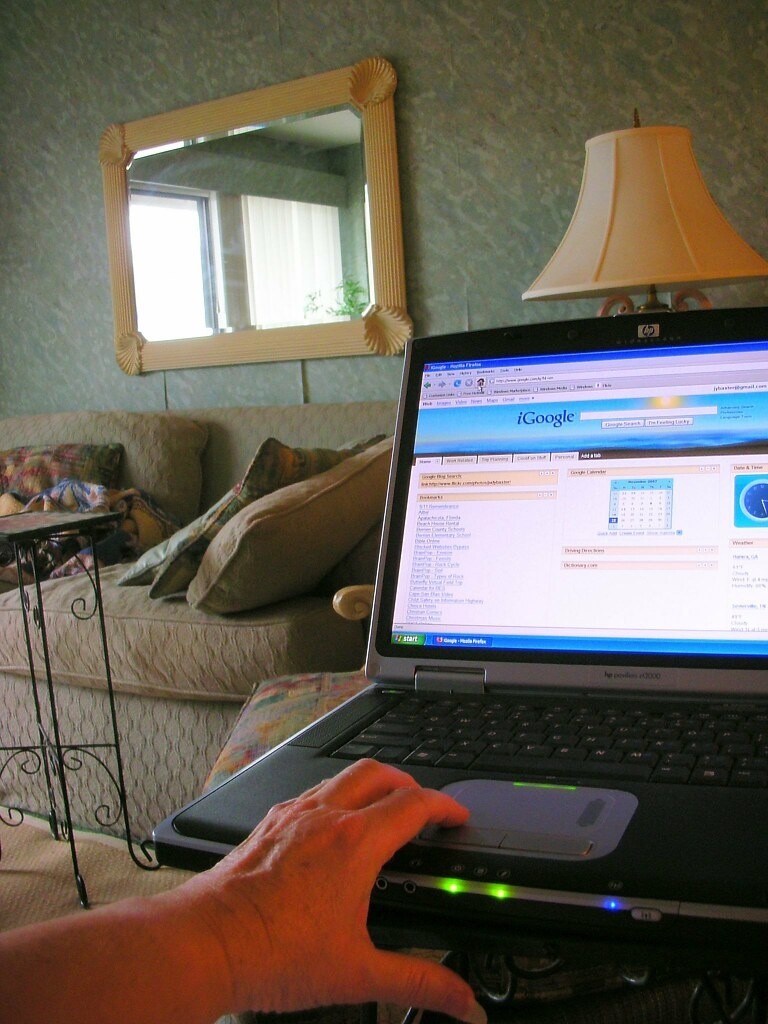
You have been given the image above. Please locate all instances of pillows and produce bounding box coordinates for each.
[1,432,396,615]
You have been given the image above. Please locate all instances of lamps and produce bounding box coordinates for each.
[521,107,768,319]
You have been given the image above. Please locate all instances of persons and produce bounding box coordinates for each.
[0,756,486,1023]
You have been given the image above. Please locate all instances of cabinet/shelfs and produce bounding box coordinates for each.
[1,512,169,909]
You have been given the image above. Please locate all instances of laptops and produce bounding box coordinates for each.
[153,304,768,954]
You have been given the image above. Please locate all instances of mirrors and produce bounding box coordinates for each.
[99,57,415,376]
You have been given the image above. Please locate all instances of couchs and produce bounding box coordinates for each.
[0,398,402,849]
